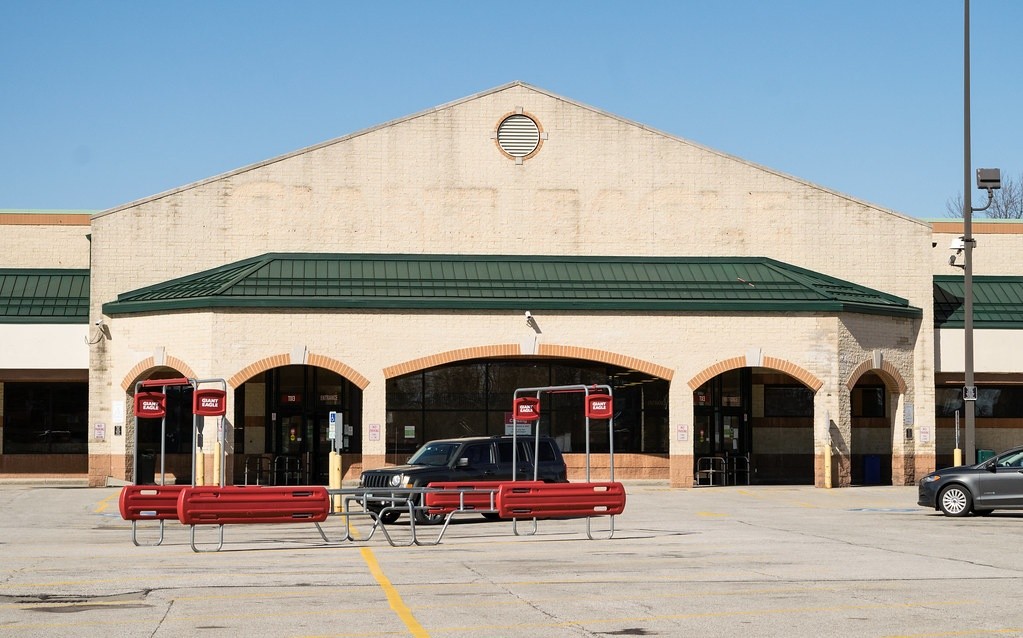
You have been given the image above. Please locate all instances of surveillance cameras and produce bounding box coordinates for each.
[948,255,956,265]
[524,311,532,320]
[94,320,104,327]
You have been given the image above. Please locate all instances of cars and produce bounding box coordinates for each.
[917,445,1023,517]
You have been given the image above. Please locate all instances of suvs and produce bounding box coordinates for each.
[356,435,569,525]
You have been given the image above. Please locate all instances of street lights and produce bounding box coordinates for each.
[949,168,1001,465]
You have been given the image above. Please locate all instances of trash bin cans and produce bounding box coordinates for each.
[862,454,882,485]
[976,450,996,466]
